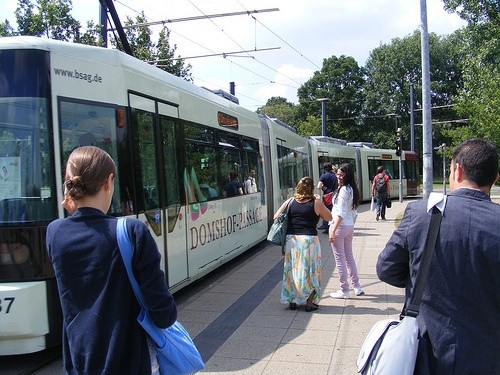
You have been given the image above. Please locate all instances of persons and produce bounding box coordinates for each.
[273,177,332,312]
[244,168,257,195]
[46,146,177,375]
[223,172,243,198]
[317,162,337,234]
[376,138,500,375]
[79,134,112,154]
[327,163,364,299]
[371,166,391,221]
[199,175,218,198]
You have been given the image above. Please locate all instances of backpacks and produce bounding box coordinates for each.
[376,174,386,193]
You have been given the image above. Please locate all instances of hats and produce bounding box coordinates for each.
[323,162,334,170]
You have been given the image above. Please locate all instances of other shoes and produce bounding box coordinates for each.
[376,213,380,221]
[322,229,328,234]
[382,218,386,220]
[317,225,329,230]
[290,303,297,310]
[306,303,319,312]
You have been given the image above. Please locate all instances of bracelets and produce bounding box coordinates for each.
[372,193,374,194]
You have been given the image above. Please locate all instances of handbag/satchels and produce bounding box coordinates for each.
[323,191,334,206]
[267,212,288,246]
[387,192,391,208]
[137,304,205,375]
[356,315,420,375]
[370,195,375,211]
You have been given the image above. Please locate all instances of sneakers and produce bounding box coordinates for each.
[330,290,350,299]
[354,288,366,296]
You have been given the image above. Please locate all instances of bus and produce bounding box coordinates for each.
[0,35,419,356]
